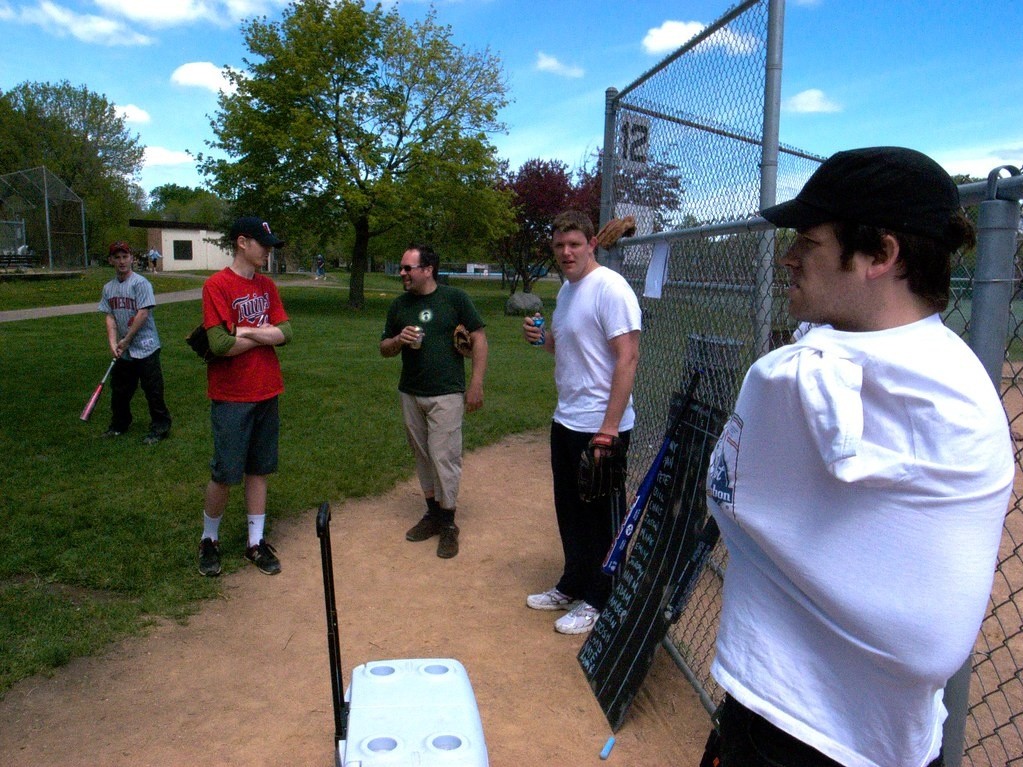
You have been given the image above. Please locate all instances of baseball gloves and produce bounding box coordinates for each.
[184,320,230,361]
[453,325,472,358]
[596,216,637,249]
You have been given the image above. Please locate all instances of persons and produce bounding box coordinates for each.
[394,262,399,276]
[136,246,164,274]
[379,242,489,558]
[524,210,642,634]
[97,240,172,445]
[0,245,31,255]
[197,217,293,577]
[697,146,1015,767]
[315,255,327,280]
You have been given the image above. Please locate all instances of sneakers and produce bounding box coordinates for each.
[199,536,222,576]
[406,509,442,541]
[527,589,584,611]
[554,600,600,635]
[245,539,281,574]
[438,524,459,558]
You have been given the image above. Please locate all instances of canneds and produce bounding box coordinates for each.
[410,327,423,349]
[529,317,545,346]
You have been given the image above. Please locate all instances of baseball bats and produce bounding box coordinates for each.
[79,355,119,423]
[599,360,704,578]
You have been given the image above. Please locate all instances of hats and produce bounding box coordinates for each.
[760,145,961,230]
[229,217,284,250]
[109,241,131,256]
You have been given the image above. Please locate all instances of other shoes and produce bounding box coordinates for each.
[154,271,157,274]
[102,430,128,437]
[143,429,170,444]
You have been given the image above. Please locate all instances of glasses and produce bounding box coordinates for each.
[399,265,424,271]
